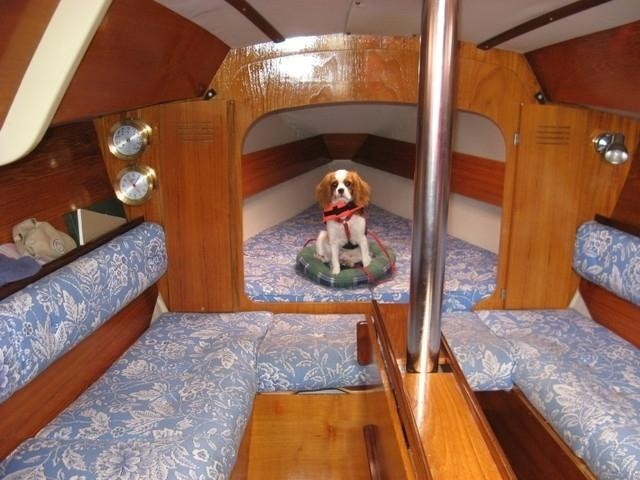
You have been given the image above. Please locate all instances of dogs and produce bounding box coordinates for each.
[313,169,373,274]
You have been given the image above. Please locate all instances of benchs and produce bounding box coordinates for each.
[0,221,268,480]
[474,218,640,480]
[258,311,513,408]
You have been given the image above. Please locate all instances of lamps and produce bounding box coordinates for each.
[594,132,627,167]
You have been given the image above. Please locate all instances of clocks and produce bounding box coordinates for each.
[106,119,159,208]
